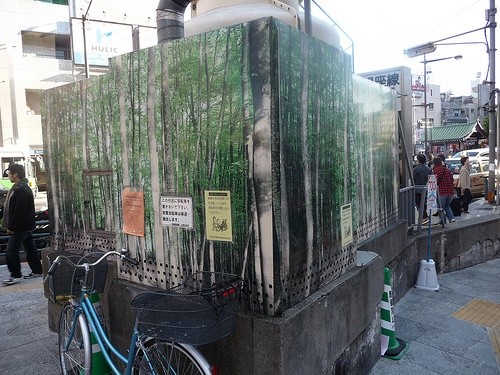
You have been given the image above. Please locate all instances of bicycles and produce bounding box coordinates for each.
[41,247,244,375]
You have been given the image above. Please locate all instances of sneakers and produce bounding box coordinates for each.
[432,212,438,216]
[3,276,24,285]
[24,272,43,279]
[422,217,429,224]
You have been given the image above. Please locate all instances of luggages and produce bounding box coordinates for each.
[450,197,462,216]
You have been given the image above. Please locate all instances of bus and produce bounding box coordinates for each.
[0,144,44,198]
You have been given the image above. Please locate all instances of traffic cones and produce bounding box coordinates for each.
[379,267,410,361]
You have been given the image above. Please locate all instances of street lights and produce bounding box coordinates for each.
[411,53,463,156]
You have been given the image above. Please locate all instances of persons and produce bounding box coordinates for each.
[2,163,43,284]
[413,154,456,223]
[457,157,472,213]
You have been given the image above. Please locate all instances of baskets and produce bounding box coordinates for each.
[47,251,109,303]
[131,270,243,346]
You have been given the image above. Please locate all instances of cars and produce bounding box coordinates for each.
[0,184,50,257]
[443,147,500,200]
